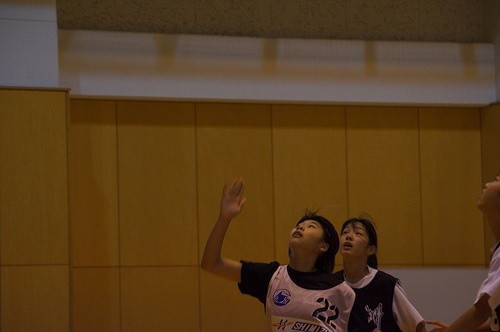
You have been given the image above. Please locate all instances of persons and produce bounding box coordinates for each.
[199,176,369,332]
[424,171,500,331]
[332,210,426,332]
